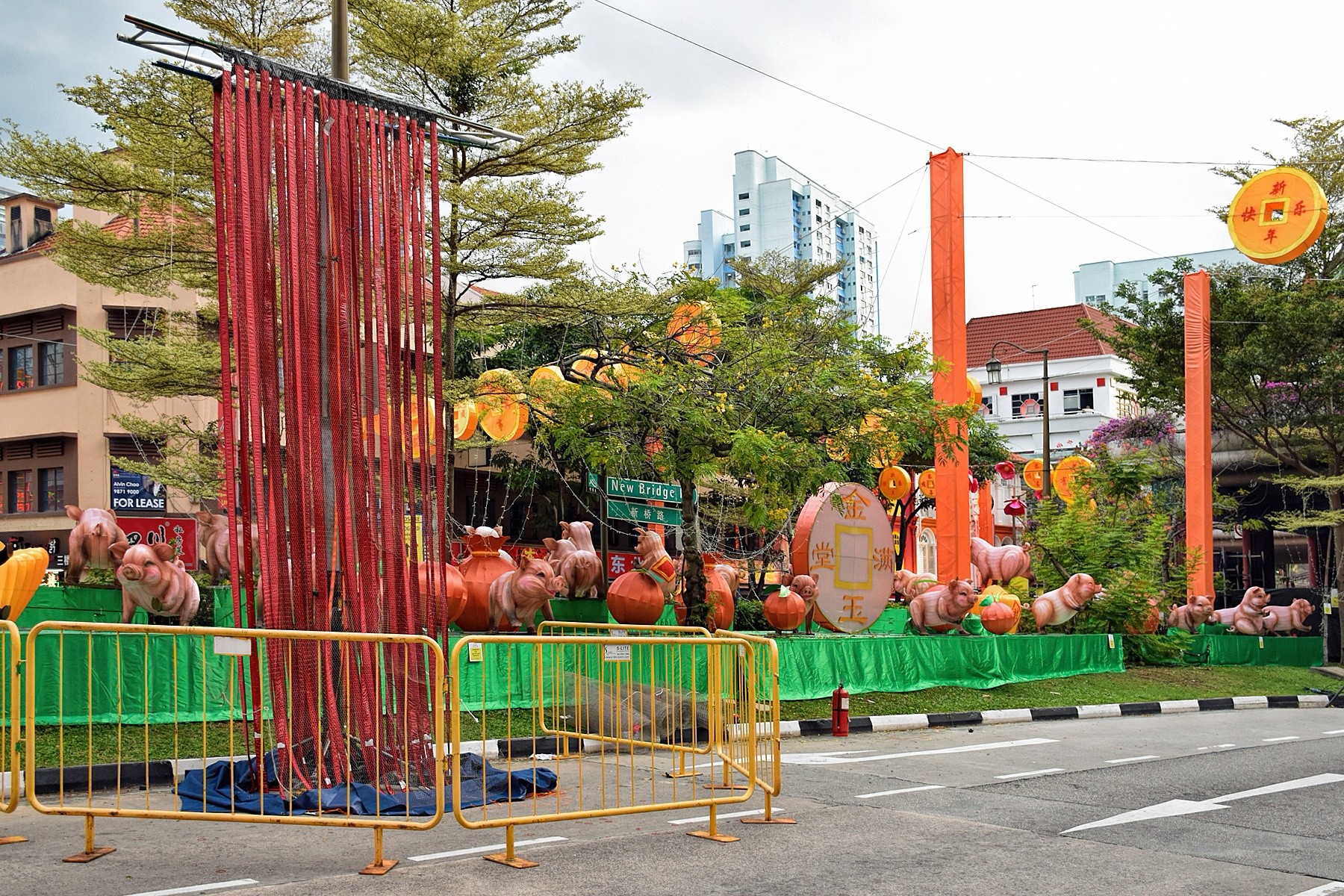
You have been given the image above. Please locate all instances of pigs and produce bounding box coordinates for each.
[61,503,264,626]
[892,537,1102,637]
[1166,585,1316,637]
[487,521,820,635]
